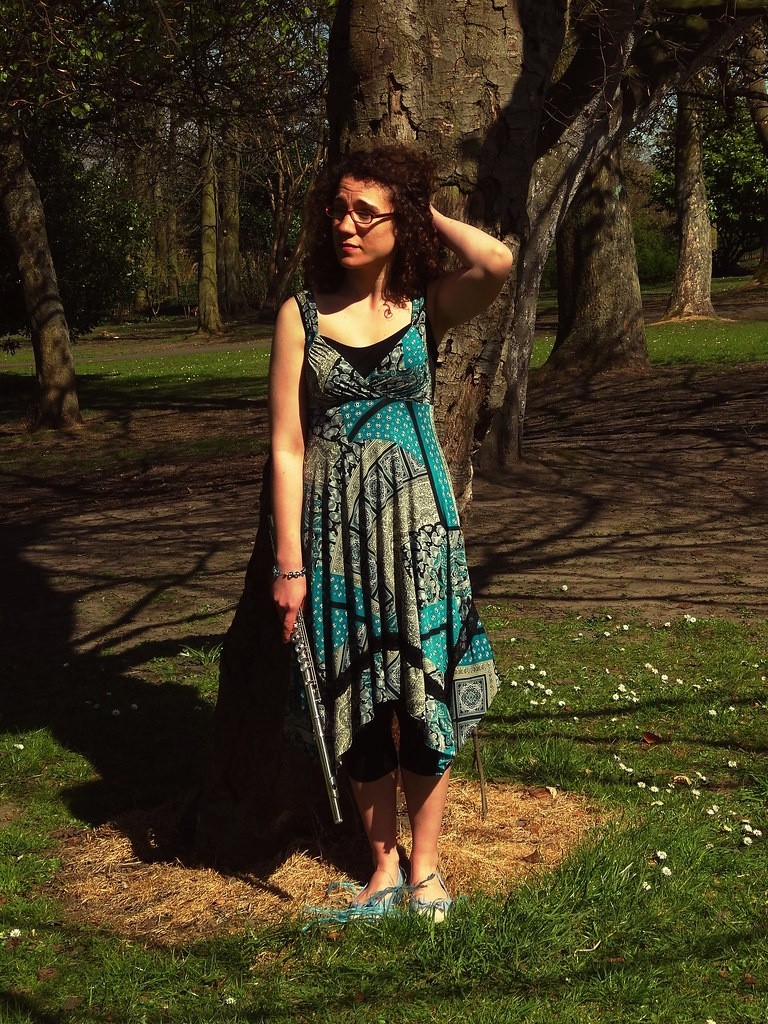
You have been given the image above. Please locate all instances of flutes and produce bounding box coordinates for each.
[265,513,344,826]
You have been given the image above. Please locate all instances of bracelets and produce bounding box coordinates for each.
[272,565,307,580]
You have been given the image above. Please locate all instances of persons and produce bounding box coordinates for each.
[268,142,514,923]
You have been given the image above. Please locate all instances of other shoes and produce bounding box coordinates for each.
[409,870,451,924]
[350,867,403,923]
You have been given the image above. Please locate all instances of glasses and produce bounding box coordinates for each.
[326,205,395,224]
